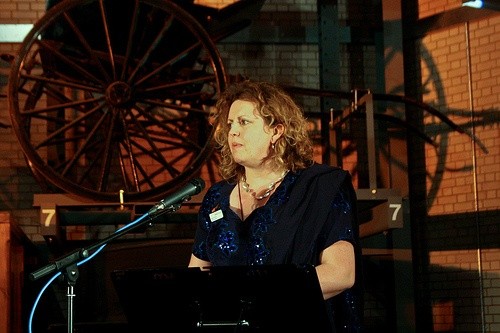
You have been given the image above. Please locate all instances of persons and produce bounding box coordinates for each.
[187,80,362,333]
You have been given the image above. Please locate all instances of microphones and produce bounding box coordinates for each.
[148,178,206,215]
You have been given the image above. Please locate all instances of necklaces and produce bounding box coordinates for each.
[241,170,290,200]
